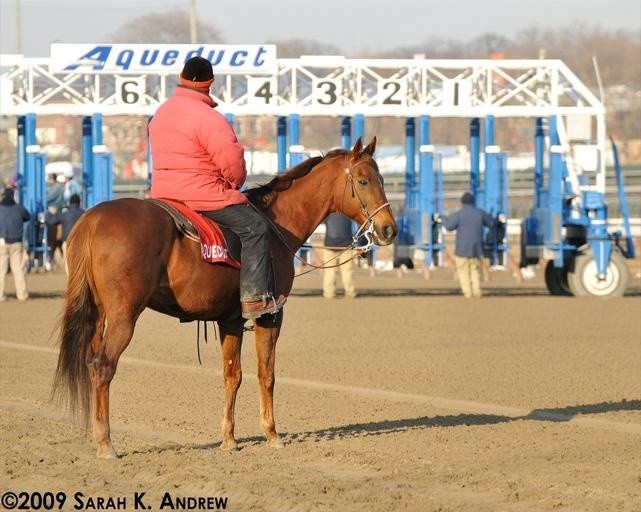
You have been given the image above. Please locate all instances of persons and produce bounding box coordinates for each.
[440,191,498,299]
[1,170,89,301]
[147,57,287,319]
[322,210,361,297]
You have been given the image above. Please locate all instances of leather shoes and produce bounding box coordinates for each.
[242,294,287,319]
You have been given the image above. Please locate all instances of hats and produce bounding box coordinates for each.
[180,56,215,88]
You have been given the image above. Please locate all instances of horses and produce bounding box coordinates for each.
[50,135,399,461]
